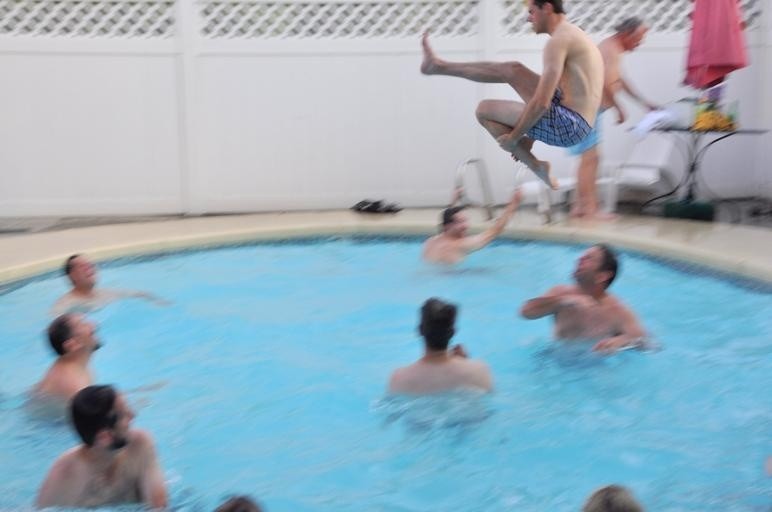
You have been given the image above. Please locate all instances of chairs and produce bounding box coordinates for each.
[516,101,698,213]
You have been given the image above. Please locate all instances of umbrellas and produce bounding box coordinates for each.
[683,0,752,110]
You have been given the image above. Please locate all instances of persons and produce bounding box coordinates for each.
[419,186,524,273]
[32,386,170,511]
[46,253,174,319]
[26,313,154,425]
[569,16,664,219]
[388,297,494,395]
[519,243,667,357]
[420,0,606,191]
